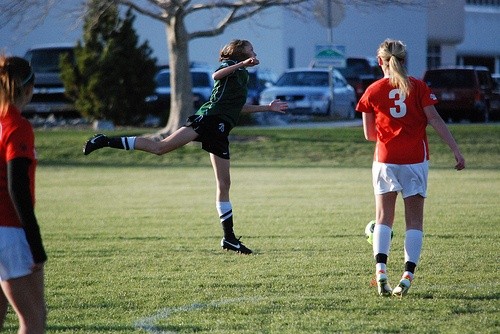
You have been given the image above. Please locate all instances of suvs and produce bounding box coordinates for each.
[22,45,84,115]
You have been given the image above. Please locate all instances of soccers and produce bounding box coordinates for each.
[364,220,394,245]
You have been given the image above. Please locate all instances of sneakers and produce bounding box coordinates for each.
[392,279,411,298]
[376,274,393,298]
[220,237,252,255]
[83,133,104,156]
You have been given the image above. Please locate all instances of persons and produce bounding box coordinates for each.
[82,39,288,255]
[0,55,49,334]
[355,40,465,296]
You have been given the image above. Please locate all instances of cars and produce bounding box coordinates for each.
[143,67,214,108]
[422,66,496,123]
[259,67,357,120]
